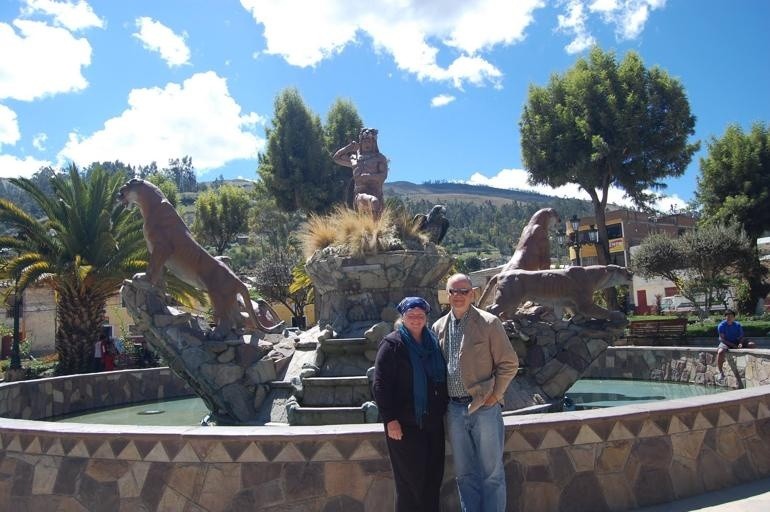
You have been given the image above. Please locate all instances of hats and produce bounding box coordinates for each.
[396,297,430,315]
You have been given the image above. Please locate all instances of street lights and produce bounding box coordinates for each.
[10,229,28,369]
[557,214,599,267]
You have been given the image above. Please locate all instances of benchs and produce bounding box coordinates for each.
[622,318,690,342]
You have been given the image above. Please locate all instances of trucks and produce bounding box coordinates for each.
[660,292,736,314]
[99,325,141,371]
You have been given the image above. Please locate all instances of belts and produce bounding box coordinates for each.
[450,396,472,403]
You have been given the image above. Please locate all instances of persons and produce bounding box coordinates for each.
[716,308,756,381]
[333,127,388,224]
[371,295,450,512]
[100,339,115,372]
[429,273,521,511]
[94,332,106,371]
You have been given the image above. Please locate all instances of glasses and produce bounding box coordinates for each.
[447,288,472,296]
